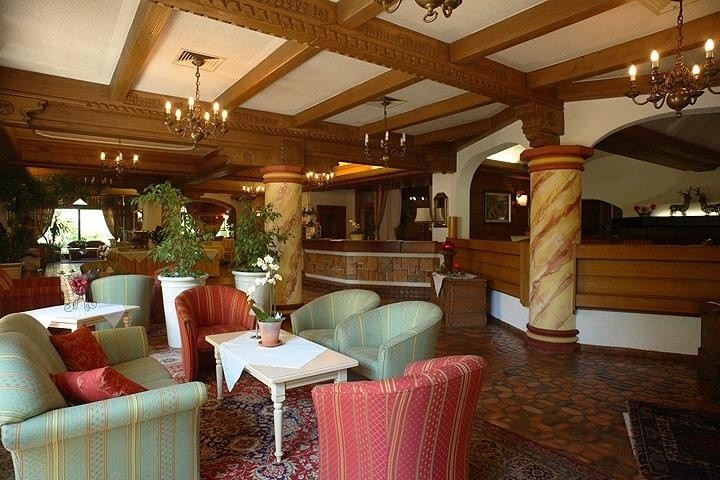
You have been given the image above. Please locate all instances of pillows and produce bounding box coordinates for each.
[50,324,112,371]
[49,365,148,402]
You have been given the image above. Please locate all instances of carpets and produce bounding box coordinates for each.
[625,396,720,479]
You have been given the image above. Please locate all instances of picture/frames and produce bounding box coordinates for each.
[433,192,448,228]
[483,189,512,225]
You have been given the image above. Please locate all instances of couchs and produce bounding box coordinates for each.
[175,285,257,381]
[0,266,64,320]
[311,355,486,479]
[0,312,207,480]
[90,274,155,332]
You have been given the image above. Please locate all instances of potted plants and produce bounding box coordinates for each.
[129,181,210,348]
[0,225,32,280]
[231,197,295,316]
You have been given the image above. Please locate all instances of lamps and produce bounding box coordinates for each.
[414,208,432,222]
[241,184,264,199]
[305,170,335,188]
[99,151,140,176]
[625,0,720,120]
[163,56,228,146]
[363,101,406,162]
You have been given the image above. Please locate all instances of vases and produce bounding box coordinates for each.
[256,320,282,348]
[350,234,364,240]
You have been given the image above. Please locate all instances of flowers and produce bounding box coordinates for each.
[348,218,365,234]
[246,253,283,322]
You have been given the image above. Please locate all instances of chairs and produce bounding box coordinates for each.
[337,301,443,380]
[290,289,380,351]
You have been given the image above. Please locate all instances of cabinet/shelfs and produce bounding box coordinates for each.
[302,238,438,300]
[444,237,720,362]
[428,271,487,329]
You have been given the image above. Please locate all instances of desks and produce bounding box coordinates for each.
[107,247,220,278]
